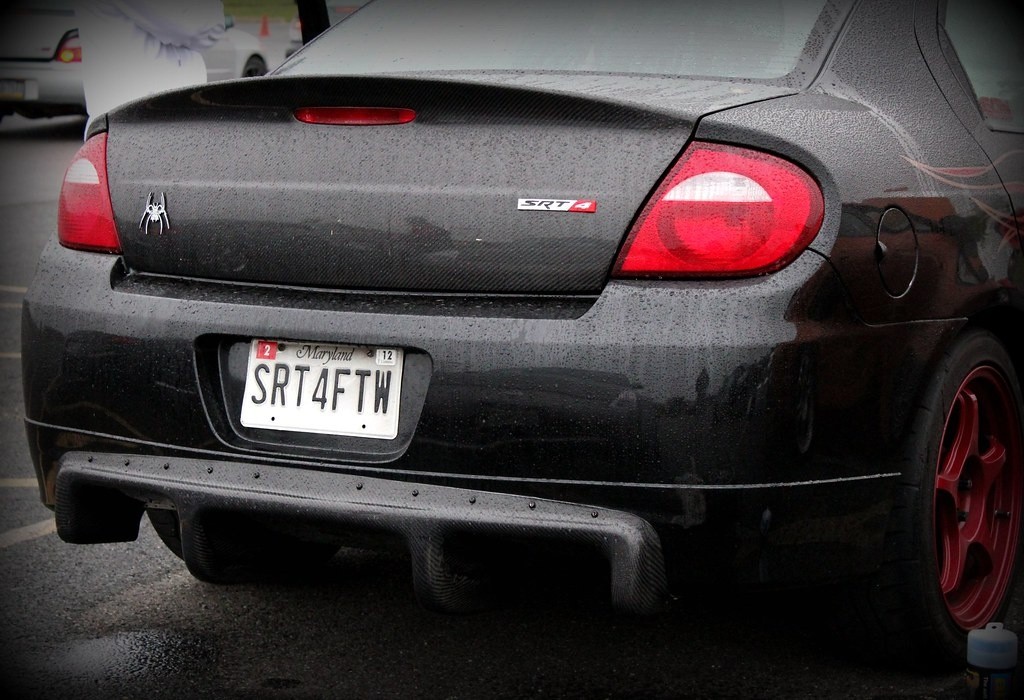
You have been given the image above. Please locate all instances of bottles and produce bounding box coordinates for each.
[965,622,1018,700]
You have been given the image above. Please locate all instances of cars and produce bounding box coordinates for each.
[0,0,298,121]
[13,0,1024,678]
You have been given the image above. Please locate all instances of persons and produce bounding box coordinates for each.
[77,0,230,142]
